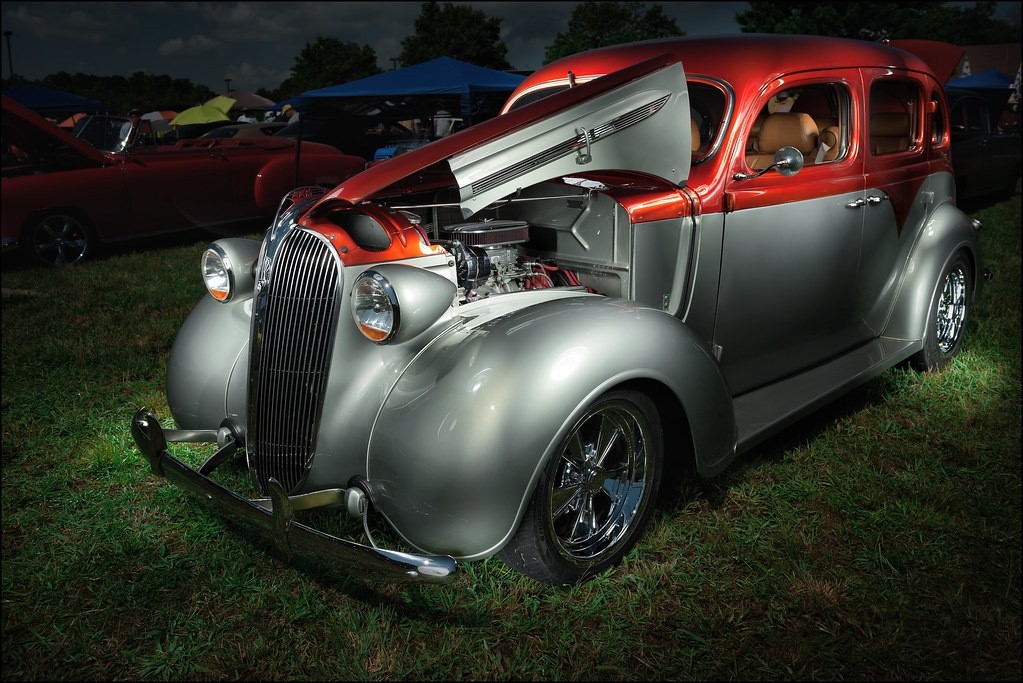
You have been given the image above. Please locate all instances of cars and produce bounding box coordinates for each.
[130,33,1023,585]
[0,94,367,268]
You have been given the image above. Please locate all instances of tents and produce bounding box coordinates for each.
[945,69,1023,134]
[267,57,527,190]
[7,83,103,133]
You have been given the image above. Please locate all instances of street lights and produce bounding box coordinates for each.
[3,30,13,80]
[225,78,232,93]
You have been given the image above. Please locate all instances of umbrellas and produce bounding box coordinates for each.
[45,113,90,128]
[138,92,277,146]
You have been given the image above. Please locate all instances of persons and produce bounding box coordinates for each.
[119,112,143,144]
[281,104,300,126]
[237,107,259,124]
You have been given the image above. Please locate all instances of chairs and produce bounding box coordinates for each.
[690,108,912,169]
